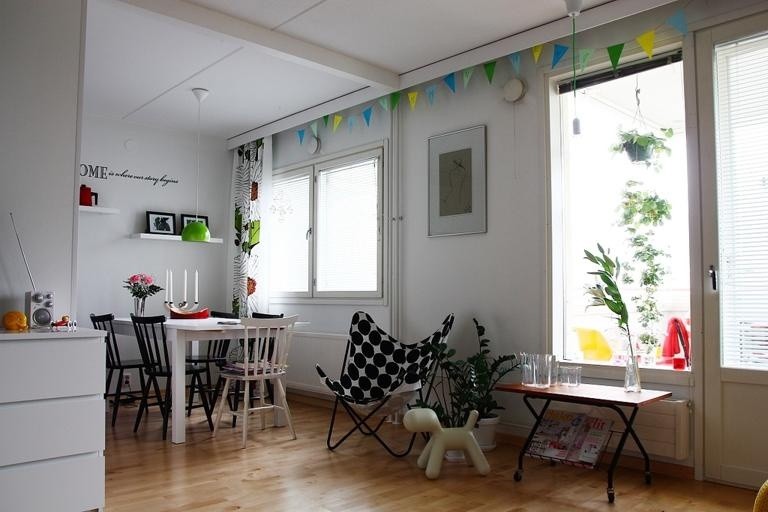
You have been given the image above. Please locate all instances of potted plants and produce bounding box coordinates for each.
[409,316,529,452]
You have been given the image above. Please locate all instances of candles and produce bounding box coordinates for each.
[165,269,198,302]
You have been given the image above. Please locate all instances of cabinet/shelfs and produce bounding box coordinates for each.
[0,326,107,512]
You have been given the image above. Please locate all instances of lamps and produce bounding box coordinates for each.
[183,88,210,243]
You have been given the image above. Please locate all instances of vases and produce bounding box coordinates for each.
[134,298,145,317]
[622,335,642,393]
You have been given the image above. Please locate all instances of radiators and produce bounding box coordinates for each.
[543,397,691,461]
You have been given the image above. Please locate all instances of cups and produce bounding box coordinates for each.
[521,353,582,389]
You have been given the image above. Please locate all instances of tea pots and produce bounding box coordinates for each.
[80,183,98,207]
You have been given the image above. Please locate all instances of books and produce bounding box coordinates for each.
[223,360,288,375]
[526,408,616,471]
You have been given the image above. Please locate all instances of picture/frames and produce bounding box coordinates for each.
[146,212,208,234]
[427,125,487,237]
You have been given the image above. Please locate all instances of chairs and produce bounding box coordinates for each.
[315,309,453,458]
[89,311,300,449]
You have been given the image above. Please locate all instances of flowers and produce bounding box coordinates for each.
[582,242,638,380]
[122,272,165,299]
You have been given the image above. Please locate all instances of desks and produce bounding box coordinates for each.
[495,382,672,504]
